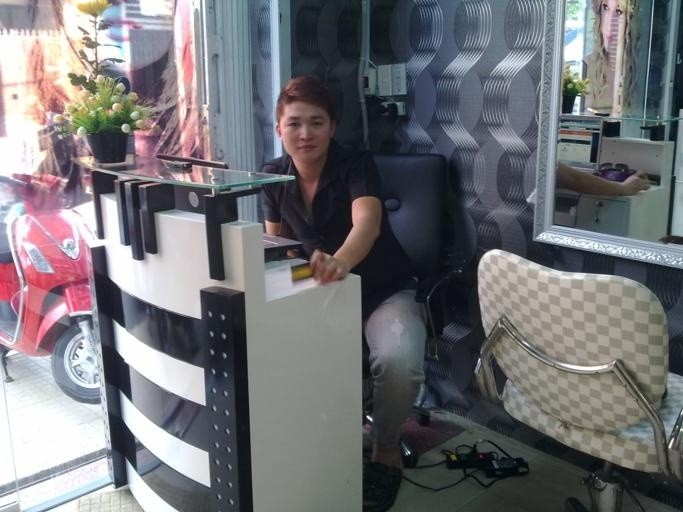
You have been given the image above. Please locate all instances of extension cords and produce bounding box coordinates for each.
[446,453,495,469]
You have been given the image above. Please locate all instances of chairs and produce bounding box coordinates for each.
[475,248,682,511]
[363,154,465,466]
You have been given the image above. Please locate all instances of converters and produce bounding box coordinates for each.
[486,458,519,478]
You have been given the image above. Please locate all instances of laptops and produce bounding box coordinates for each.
[557,114,603,175]
[174,185,304,265]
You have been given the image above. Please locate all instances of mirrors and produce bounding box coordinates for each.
[530,0,683,273]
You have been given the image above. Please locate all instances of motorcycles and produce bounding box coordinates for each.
[0,171,103,405]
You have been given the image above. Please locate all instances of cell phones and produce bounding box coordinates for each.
[595,113,609,117]
[167,160,192,168]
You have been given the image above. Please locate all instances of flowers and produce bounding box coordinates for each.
[560,61,590,97]
[54,1,152,136]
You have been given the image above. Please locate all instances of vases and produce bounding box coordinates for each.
[85,130,127,163]
[562,95,575,113]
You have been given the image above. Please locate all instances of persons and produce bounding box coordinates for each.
[586,1,643,112]
[257,71,430,511]
[556,160,652,196]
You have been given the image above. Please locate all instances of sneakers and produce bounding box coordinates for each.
[362,460,403,512]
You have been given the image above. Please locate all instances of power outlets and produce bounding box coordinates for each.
[364,63,407,117]
